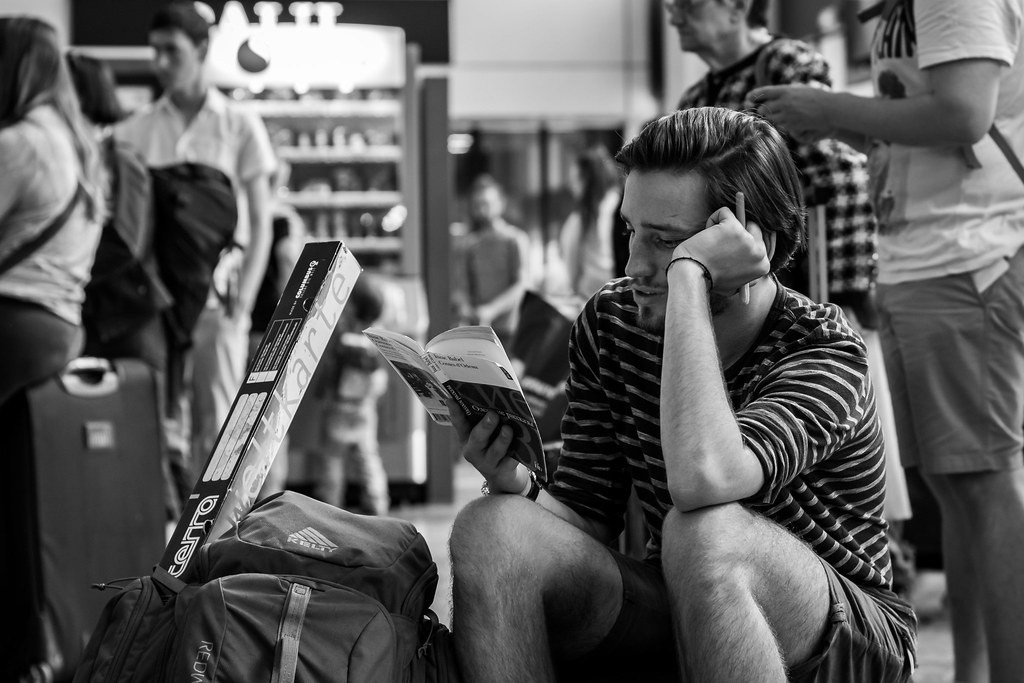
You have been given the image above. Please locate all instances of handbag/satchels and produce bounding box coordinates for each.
[144,161,237,411]
[82,221,171,355]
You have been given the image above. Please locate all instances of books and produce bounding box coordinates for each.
[362,324,549,485]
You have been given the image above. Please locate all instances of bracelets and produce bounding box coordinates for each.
[665,257,715,293]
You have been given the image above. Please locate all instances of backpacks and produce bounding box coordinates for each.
[73,490,454,683]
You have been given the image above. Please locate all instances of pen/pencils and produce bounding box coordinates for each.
[735,190,751,305]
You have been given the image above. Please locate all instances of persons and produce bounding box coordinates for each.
[0,15,130,683]
[104,12,277,500]
[663,0,1024,683]
[561,142,625,314]
[451,174,530,344]
[312,288,391,525]
[450,106,918,682]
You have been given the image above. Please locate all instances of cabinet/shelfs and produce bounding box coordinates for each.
[255,96,403,255]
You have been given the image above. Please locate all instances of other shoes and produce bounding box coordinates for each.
[909,570,945,615]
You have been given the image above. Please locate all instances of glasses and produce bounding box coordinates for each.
[662,0,713,18]
